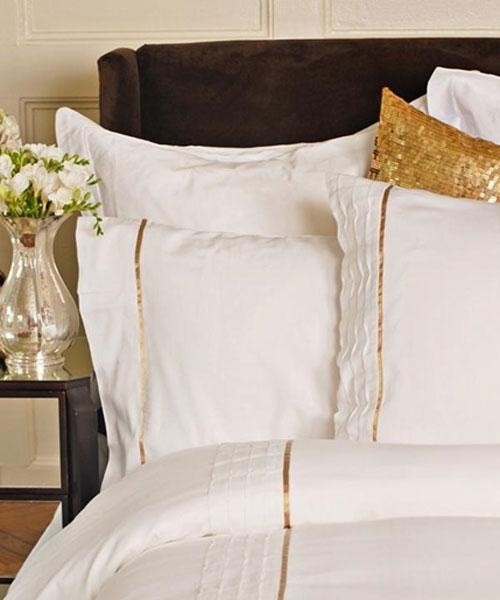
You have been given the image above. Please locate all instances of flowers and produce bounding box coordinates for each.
[0,114,105,243]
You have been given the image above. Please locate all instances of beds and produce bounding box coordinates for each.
[7,32,500,600]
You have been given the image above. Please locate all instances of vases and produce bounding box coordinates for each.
[0,215,80,373]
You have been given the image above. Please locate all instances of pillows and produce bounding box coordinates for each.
[73,211,341,495]
[368,87,500,200]
[319,166,498,446]
[425,67,496,146]
[55,95,437,239]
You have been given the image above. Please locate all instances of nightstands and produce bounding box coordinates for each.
[0,366,101,527]
